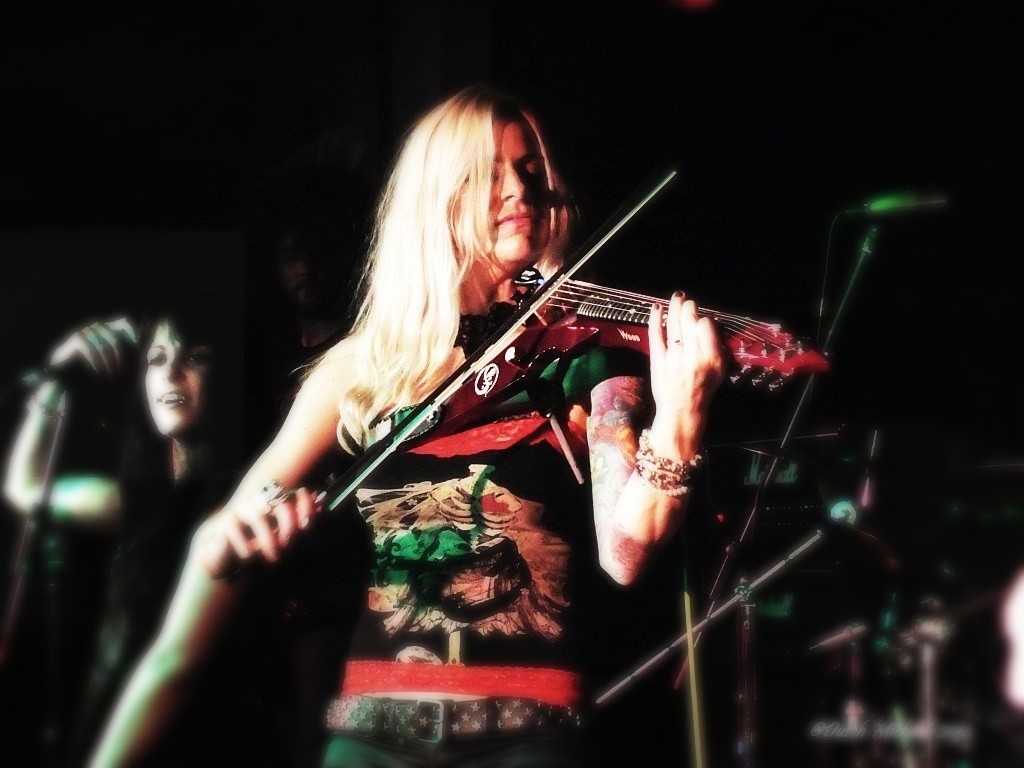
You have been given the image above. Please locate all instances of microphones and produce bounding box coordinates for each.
[23,334,138,390]
[855,186,972,216]
[828,503,858,526]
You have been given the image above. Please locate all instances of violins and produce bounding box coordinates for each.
[369,277,838,454]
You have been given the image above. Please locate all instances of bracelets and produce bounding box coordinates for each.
[29,398,62,420]
[634,424,709,495]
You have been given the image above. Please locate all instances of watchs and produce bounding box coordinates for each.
[22,357,62,393]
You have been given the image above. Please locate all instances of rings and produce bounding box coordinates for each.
[259,484,290,508]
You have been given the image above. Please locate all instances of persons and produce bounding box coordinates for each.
[217,86,728,767]
[0,214,364,768]
